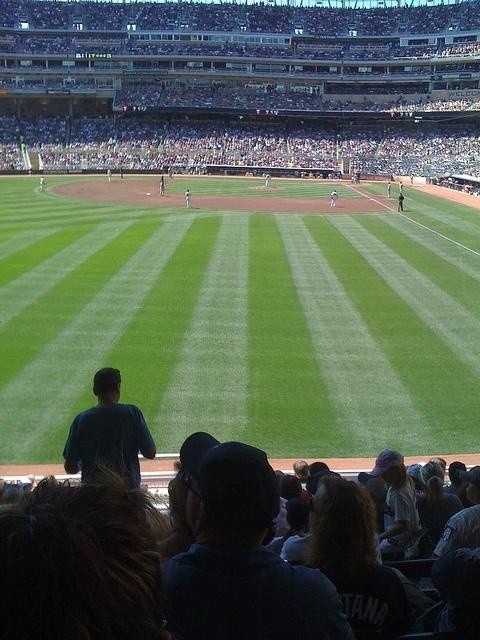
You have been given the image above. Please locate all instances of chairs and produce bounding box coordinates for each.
[284,545,445,640]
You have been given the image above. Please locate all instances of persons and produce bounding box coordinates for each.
[185,189,192,208]
[331,190,338,207]
[398,193,404,211]
[63,368,156,489]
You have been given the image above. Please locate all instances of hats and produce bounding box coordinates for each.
[456,466,480,484]
[180,431,281,521]
[369,448,404,478]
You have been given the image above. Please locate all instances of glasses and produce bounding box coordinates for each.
[180,470,215,516]
